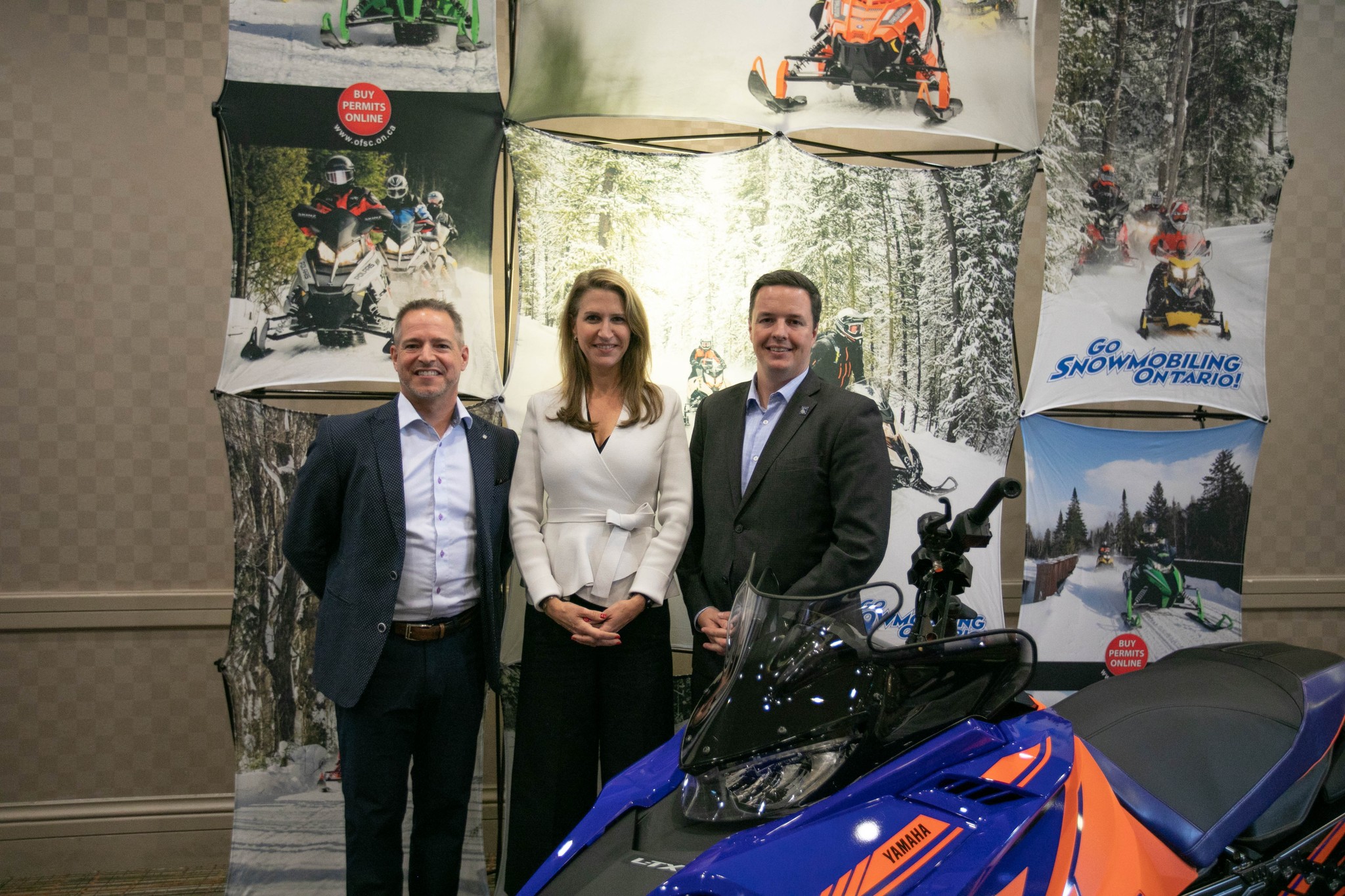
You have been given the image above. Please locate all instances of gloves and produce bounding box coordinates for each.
[692,360,698,365]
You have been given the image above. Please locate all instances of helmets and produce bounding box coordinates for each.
[1098,165,1115,187]
[834,307,864,342]
[1143,519,1156,533]
[325,155,356,188]
[386,175,408,199]
[427,191,443,205]
[1151,191,1163,207]
[700,337,711,350]
[1169,201,1190,231]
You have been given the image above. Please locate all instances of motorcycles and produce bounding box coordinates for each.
[1096,549,1116,567]
[747,0,965,120]
[1138,230,1232,339]
[842,377,958,497]
[682,358,728,427]
[240,203,461,361]
[1079,181,1137,268]
[511,474,1345,896]
[1123,538,1235,630]
[320,0,489,51]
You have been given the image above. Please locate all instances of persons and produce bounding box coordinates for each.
[280,299,519,896]
[1098,540,1112,554]
[509,268,694,894]
[378,175,433,232]
[1084,166,1129,208]
[425,191,459,241]
[1148,201,1211,258]
[1133,190,1167,223]
[297,157,387,237]
[675,271,890,708]
[1129,521,1176,556]
[809,307,873,394]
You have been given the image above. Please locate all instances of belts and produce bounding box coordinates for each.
[386,612,474,642]
